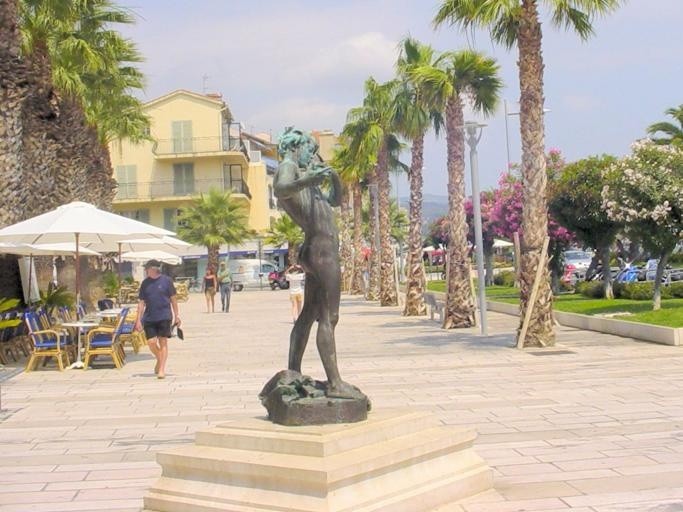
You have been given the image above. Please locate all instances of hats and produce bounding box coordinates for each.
[141,259,160,267]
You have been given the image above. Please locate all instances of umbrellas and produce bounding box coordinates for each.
[0,201,192,316]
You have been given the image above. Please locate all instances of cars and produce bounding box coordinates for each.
[229,258,282,292]
[614,259,683,282]
[562,251,592,270]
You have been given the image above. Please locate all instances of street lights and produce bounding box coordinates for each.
[456,120,489,336]
[503,98,553,173]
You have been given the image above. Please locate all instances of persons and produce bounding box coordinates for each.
[285,265,306,323]
[201,267,216,313]
[135,259,181,379]
[272,124,367,403]
[216,261,233,313]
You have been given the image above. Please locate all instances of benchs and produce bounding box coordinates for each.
[423,292,445,324]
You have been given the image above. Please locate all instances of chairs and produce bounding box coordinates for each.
[0,299,148,370]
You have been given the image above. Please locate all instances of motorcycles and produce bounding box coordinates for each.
[268,271,289,291]
